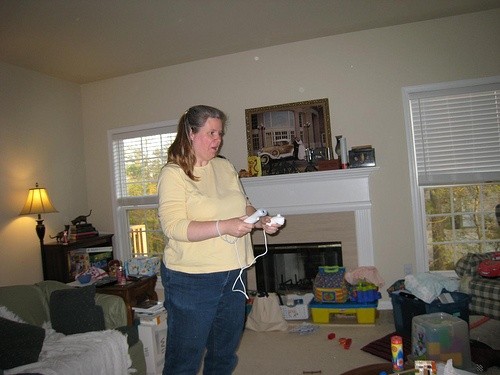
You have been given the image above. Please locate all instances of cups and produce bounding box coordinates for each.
[117,266,127,284]
[305,148,315,164]
[248,156,262,177]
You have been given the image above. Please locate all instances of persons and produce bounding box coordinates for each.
[296,137,306,160]
[155,103,280,375]
[293,137,300,161]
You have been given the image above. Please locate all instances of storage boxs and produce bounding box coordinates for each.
[391,291,471,337]
[406,310,478,373]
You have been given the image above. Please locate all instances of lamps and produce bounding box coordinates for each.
[19,182,60,281]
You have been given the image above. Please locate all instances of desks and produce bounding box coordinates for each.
[97,273,158,345]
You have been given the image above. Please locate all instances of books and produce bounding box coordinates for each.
[76,246,112,253]
[71,223,99,240]
[132,297,168,326]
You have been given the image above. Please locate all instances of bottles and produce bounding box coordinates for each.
[391,336,404,372]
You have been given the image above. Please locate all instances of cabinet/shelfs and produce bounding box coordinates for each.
[239,166,383,292]
[45,234,115,284]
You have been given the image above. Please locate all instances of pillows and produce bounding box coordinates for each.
[49,284,106,337]
[1,318,45,369]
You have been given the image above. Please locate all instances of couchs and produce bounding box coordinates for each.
[455,203,499,318]
[1,277,137,375]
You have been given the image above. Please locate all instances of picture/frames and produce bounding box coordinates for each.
[243,96,335,159]
